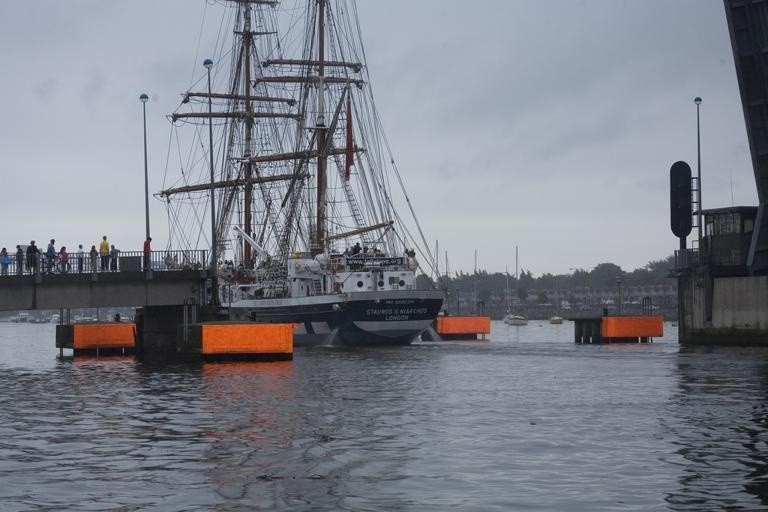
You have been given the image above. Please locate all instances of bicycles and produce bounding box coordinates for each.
[36,253,70,274]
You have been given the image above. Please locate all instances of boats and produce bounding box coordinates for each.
[551,315,563,323]
[503,314,527,325]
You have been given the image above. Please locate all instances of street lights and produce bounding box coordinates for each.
[695,96,703,253]
[140,92,150,263]
[204,57,216,299]
[616,275,622,313]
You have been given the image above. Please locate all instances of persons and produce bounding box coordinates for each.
[110,244,121,271]
[15,245,24,275]
[143,238,153,272]
[100,236,110,272]
[77,245,84,273]
[59,246,70,274]
[46,238,59,274]
[1,247,11,276]
[90,245,99,273]
[343,242,416,270]
[26,240,42,275]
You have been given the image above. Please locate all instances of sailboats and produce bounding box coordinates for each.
[153,0,448,347]
